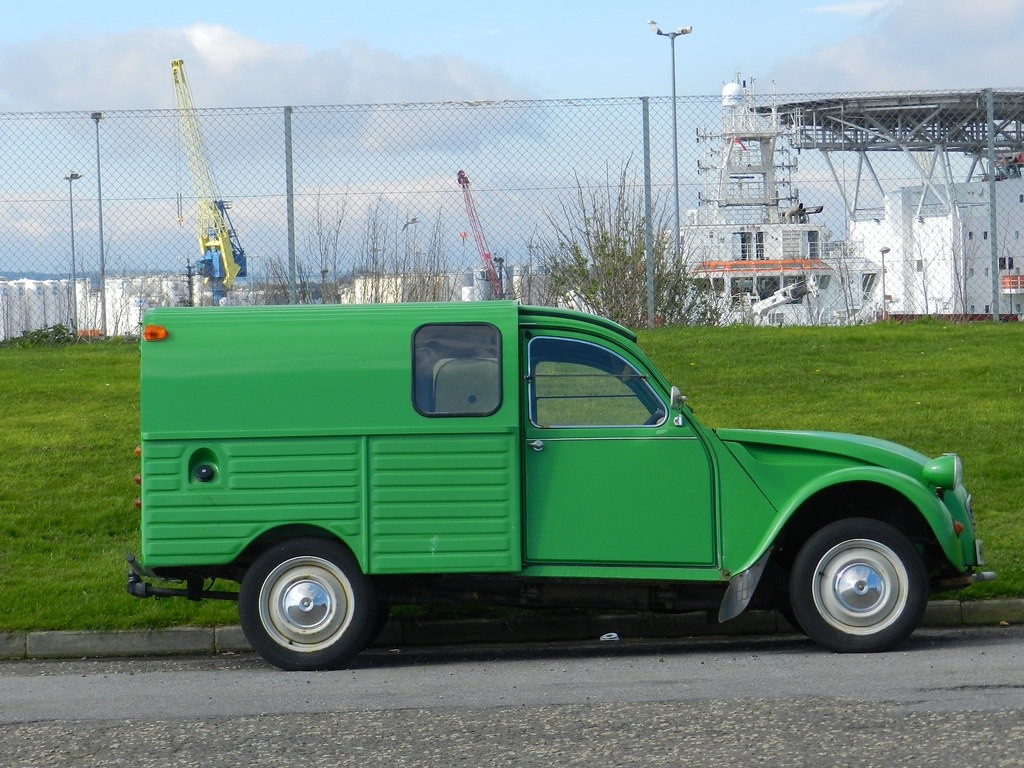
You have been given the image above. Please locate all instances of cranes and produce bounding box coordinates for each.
[457,171,506,297]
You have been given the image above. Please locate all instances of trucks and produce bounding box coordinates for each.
[127,301,996,661]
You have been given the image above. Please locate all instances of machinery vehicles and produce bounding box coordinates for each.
[169,59,250,306]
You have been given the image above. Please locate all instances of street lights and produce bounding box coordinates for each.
[646,19,695,302]
[63,170,82,338]
[90,112,113,341]
[881,247,891,322]
